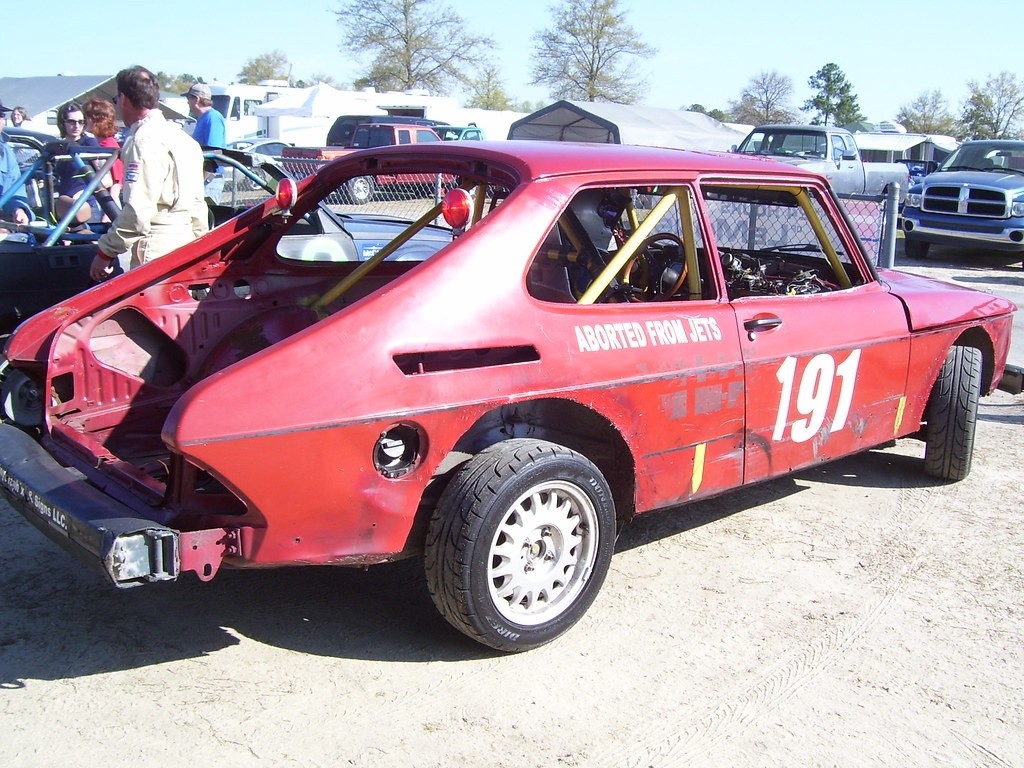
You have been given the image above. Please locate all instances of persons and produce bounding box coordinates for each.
[0,101,37,243]
[82,99,122,222]
[91,66,210,283]
[47,100,113,223]
[180,82,226,206]
[9,108,41,163]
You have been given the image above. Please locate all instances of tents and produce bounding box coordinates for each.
[255,82,388,148]
[0,76,197,132]
[506,100,749,152]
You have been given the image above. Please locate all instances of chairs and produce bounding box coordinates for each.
[969,158,994,171]
[527,193,630,303]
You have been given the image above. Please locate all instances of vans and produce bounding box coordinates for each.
[325,114,450,146]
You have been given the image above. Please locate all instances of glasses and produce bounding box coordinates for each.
[0,113,6,118]
[65,118,86,126]
[112,94,120,104]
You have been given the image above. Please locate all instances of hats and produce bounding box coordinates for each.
[0,104,14,111]
[180,83,212,101]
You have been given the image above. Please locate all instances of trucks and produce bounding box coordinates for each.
[181,79,304,148]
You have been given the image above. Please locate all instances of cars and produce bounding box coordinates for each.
[0,126,58,152]
[0,142,463,337]
[226,137,294,156]
[0,140,1018,656]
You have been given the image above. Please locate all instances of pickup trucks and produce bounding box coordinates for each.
[430,125,486,141]
[281,122,457,205]
[726,124,910,206]
[901,139,1024,269]
